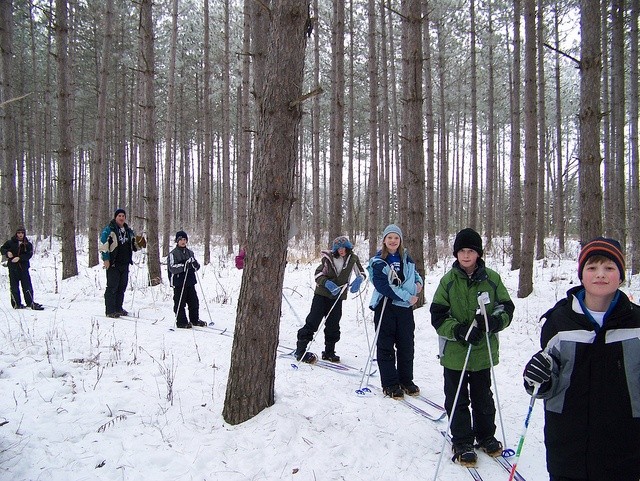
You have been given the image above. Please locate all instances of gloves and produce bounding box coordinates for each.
[523,351,553,387]
[185,257,192,272]
[350,276,364,293]
[457,323,483,346]
[324,280,341,296]
[191,256,200,271]
[474,314,500,332]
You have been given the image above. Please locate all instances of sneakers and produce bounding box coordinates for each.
[453,442,477,463]
[294,351,318,365]
[475,437,503,454]
[400,381,420,397]
[190,320,206,326]
[119,310,128,316]
[176,322,192,329]
[26,302,42,307]
[13,304,27,309]
[106,312,120,319]
[382,385,404,399]
[322,351,340,363]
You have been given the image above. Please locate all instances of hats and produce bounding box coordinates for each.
[175,231,188,243]
[16,226,26,233]
[453,228,483,258]
[383,224,403,243]
[113,208,127,219]
[332,236,352,252]
[578,238,626,281]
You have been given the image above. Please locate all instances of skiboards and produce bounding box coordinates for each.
[177,326,234,334]
[367,383,447,420]
[94,314,165,324]
[280,350,377,375]
[12,304,72,311]
[222,327,298,354]
[437,426,526,480]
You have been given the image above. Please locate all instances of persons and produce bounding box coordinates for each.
[429,228,515,465]
[235,241,250,270]
[0,227,34,310]
[293,236,366,365]
[98,208,146,319]
[522,237,640,481]
[166,231,206,329]
[364,224,424,400]
